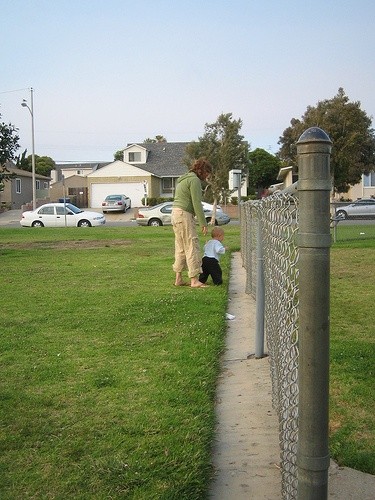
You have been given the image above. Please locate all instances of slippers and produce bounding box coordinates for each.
[192,283,209,288]
[176,281,191,286]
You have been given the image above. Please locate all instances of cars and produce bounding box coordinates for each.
[335,198,375,219]
[102,194,131,213]
[20,202,106,228]
[136,201,230,227]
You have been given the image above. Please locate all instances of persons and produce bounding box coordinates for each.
[199,227,229,286]
[171,159,213,288]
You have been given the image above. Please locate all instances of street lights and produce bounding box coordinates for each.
[20,102,36,212]
